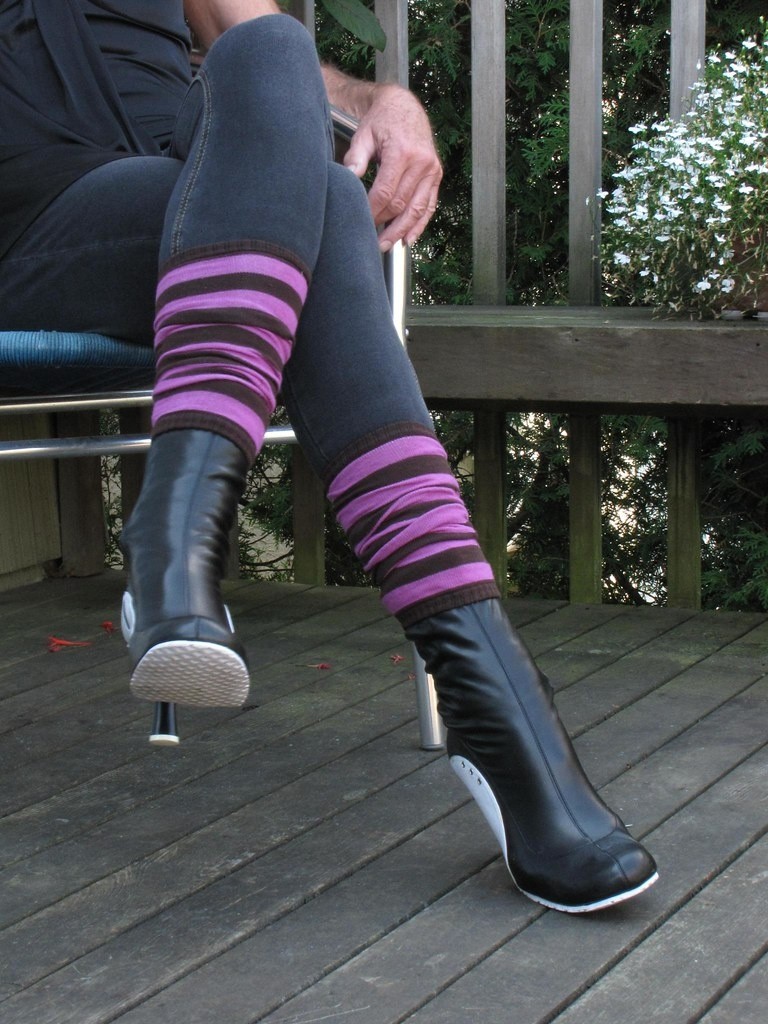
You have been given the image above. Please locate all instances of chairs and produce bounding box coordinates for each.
[0,48,449,751]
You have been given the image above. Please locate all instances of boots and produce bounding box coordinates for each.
[404,598,660,914]
[120,431,252,747]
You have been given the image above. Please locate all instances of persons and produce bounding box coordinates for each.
[1,0,661,912]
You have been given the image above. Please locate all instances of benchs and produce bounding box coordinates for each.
[379,305,768,410]
